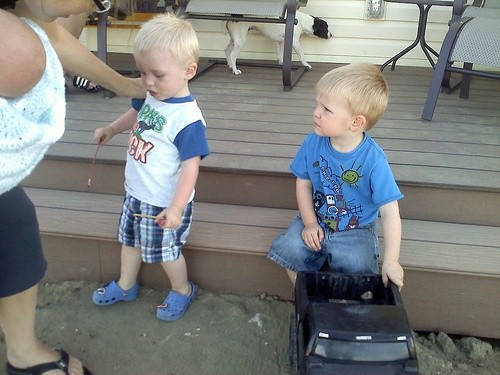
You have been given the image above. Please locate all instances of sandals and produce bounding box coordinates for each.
[92,279,139,305]
[157,281,196,321]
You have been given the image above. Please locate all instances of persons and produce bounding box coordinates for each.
[0,0,145,375]
[92,12,210,322]
[266,61,405,291]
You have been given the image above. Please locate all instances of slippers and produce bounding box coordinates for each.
[6,348,93,375]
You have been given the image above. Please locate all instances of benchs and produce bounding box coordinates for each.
[421,0,500,123]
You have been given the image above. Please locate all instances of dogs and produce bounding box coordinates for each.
[225,8,332,76]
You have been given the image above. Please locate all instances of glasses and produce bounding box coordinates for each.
[93,0,111,13]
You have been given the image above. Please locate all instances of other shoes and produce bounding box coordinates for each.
[73,75,100,92]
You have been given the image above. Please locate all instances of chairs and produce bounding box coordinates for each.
[175,0,308,91]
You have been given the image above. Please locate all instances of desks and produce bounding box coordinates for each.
[380,0,454,73]
